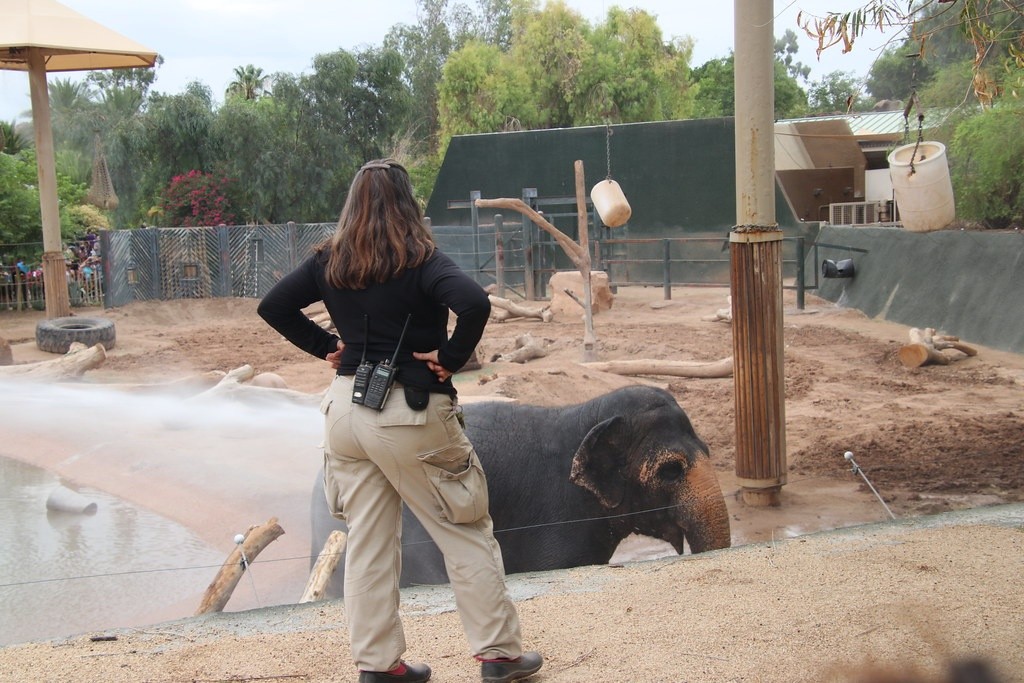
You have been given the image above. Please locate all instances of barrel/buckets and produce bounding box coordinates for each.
[590,179,632,228]
[46,486,97,513]
[888,141,956,234]
[822,259,855,279]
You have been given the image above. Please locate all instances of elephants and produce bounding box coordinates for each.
[311,385,731,600]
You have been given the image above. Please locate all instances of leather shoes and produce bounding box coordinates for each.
[481,651,543,683]
[358,660,432,683]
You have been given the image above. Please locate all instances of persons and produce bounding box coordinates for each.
[257,154,545,683]
[62,228,105,294]
[0,255,45,311]
